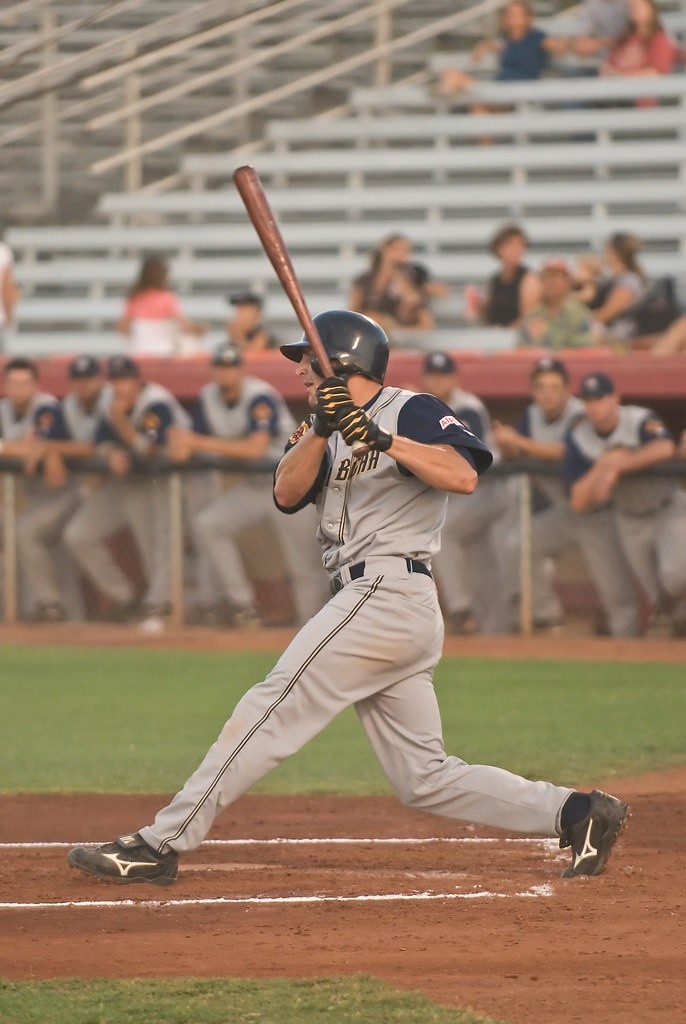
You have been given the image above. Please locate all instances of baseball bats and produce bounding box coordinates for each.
[231,164,372,459]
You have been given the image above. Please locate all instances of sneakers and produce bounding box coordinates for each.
[560,789,631,878]
[65,831,177,888]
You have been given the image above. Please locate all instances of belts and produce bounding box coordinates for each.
[330,558,433,597]
[618,496,673,518]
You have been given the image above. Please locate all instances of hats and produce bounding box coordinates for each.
[574,372,614,399]
[211,345,242,366]
[422,351,454,373]
[528,358,568,383]
[104,354,140,379]
[66,356,98,378]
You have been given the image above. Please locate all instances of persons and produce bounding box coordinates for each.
[428,0,682,147]
[66,311,634,889]
[0,203,686,637]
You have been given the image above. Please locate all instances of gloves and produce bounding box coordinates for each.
[334,406,393,453]
[313,373,354,438]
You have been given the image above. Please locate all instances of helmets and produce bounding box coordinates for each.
[280,310,389,385]
